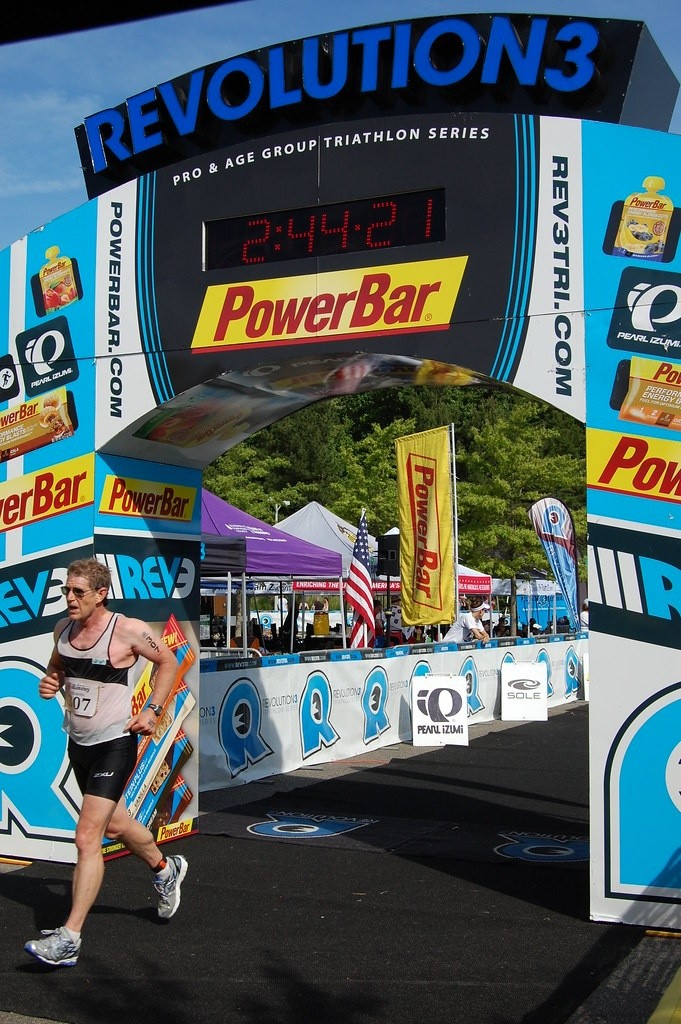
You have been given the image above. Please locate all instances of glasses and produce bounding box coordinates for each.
[60,586,97,599]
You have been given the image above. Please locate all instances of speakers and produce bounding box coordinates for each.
[376,534,401,576]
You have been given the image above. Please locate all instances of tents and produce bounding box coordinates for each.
[198,484,349,672]
[205,500,394,665]
[289,524,496,666]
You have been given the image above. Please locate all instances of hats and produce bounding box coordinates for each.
[471,602,490,612]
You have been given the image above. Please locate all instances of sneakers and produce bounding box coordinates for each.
[24,926,81,966]
[154,853,190,918]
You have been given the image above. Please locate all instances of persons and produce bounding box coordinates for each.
[21,558,191,966]
[206,587,580,671]
